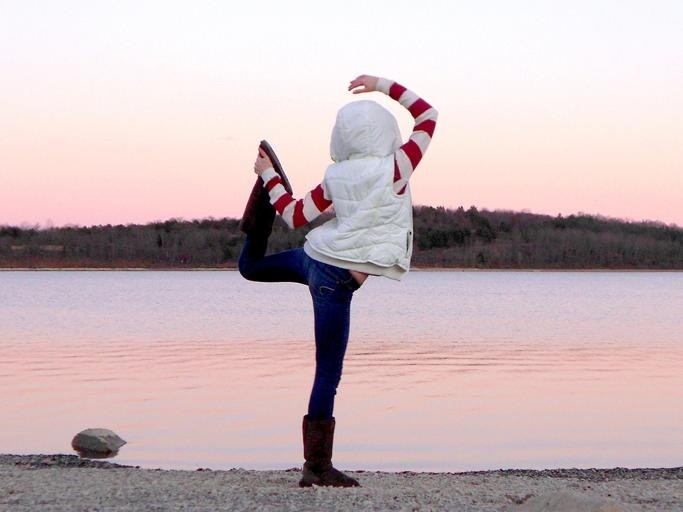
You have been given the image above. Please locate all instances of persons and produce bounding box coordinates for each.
[236,73,441,488]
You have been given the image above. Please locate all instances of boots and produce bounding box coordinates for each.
[238,141,292,237]
[299,415,359,488]
